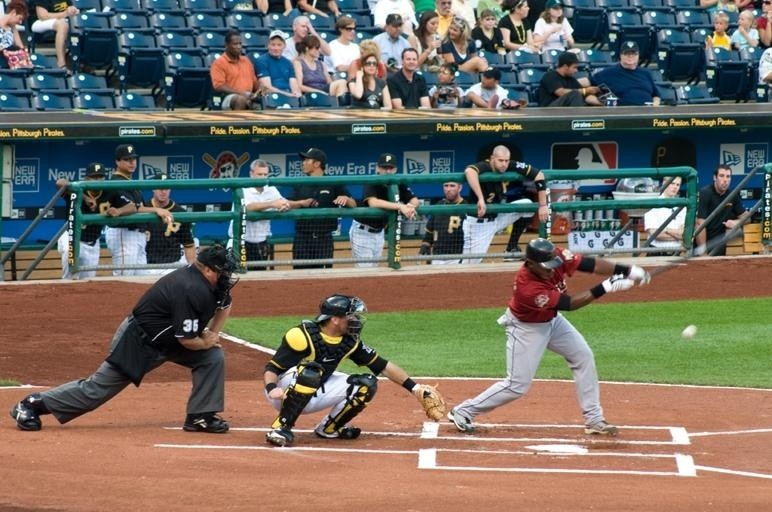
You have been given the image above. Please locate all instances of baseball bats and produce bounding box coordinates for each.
[647,228,744,279]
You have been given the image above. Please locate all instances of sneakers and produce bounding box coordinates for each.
[265,427,294,447]
[446,408,476,432]
[585,421,617,434]
[314,428,361,439]
[183,414,229,433]
[10,400,42,430]
[502,246,524,262]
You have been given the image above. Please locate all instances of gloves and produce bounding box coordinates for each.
[627,265,651,288]
[601,273,635,293]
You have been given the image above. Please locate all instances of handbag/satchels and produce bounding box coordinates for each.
[3,47,34,69]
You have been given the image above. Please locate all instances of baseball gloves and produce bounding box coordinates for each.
[413,383,445,423]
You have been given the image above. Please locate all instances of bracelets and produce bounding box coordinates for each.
[589,283,607,300]
[264,383,276,393]
[401,377,415,392]
[613,265,631,277]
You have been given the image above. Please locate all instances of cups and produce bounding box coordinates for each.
[606,98,619,106]
[572,194,621,231]
[644,103,653,107]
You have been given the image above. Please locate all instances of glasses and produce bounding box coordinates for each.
[342,26,356,32]
[365,62,378,66]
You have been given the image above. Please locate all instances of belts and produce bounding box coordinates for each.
[359,225,382,233]
[464,215,495,223]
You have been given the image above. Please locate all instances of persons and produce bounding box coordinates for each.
[1,1,772,277]
[11,242,237,430]
[446,237,654,437]
[262,293,444,448]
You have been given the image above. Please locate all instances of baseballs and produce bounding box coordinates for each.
[683,326,697,340]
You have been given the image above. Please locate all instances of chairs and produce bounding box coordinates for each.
[0,0,772,110]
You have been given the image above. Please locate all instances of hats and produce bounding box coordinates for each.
[479,66,501,80]
[86,162,107,177]
[114,144,139,160]
[299,147,327,165]
[152,173,172,179]
[378,153,397,168]
[620,41,639,53]
[269,30,286,43]
[559,51,591,65]
[545,0,563,9]
[386,14,404,26]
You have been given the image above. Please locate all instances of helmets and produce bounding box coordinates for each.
[197,246,239,289]
[314,294,368,342]
[526,237,564,270]
